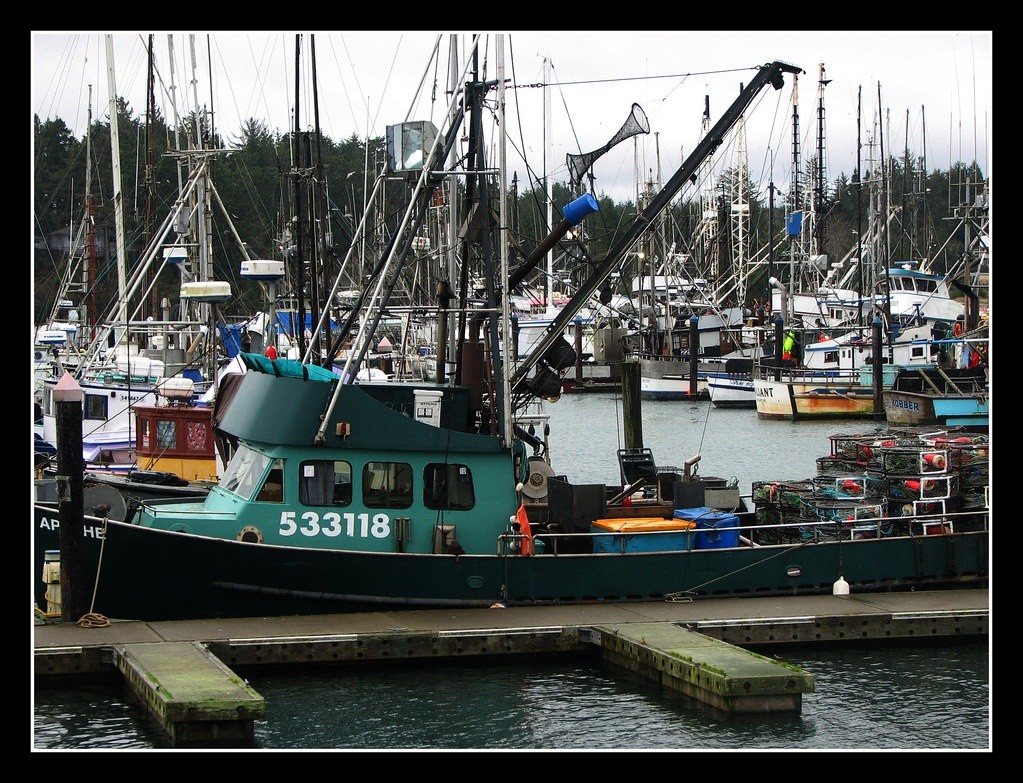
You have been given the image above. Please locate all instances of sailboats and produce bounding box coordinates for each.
[32,32,512,460]
[513,55,988,414]
[34,35,989,619]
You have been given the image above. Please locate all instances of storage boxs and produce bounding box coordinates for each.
[676,507,740,549]
[591,518,696,553]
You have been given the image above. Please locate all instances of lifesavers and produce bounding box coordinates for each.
[937,352,952,368]
[953,323,962,336]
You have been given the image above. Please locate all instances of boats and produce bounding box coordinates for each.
[882,368,989,426]
